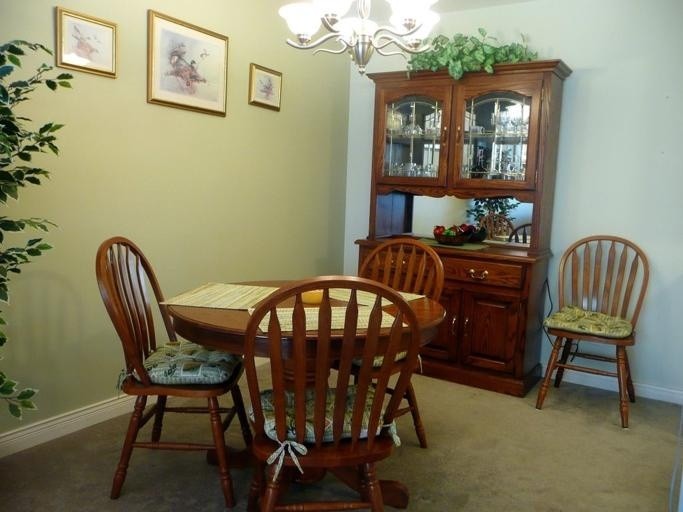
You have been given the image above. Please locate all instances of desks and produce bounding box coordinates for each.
[170,279,449,507]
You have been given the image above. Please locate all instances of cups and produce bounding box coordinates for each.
[301,290,322,304]
[383,160,525,180]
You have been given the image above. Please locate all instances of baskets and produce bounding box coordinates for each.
[434,234,466,246]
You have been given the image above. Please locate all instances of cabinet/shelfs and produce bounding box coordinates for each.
[351,233,549,398]
[360,61,574,250]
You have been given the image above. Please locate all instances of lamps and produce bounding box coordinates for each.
[275,0,444,72]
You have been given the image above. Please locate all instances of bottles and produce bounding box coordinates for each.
[386,108,527,139]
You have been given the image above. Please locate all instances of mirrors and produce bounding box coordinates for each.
[407,193,535,247]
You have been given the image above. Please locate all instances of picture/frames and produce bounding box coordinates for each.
[54,4,118,83]
[247,62,283,115]
[146,8,229,121]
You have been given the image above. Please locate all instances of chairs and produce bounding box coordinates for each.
[536,234,649,431]
[481,211,518,243]
[322,238,445,452]
[506,222,533,241]
[94,236,265,510]
[243,273,421,512]
